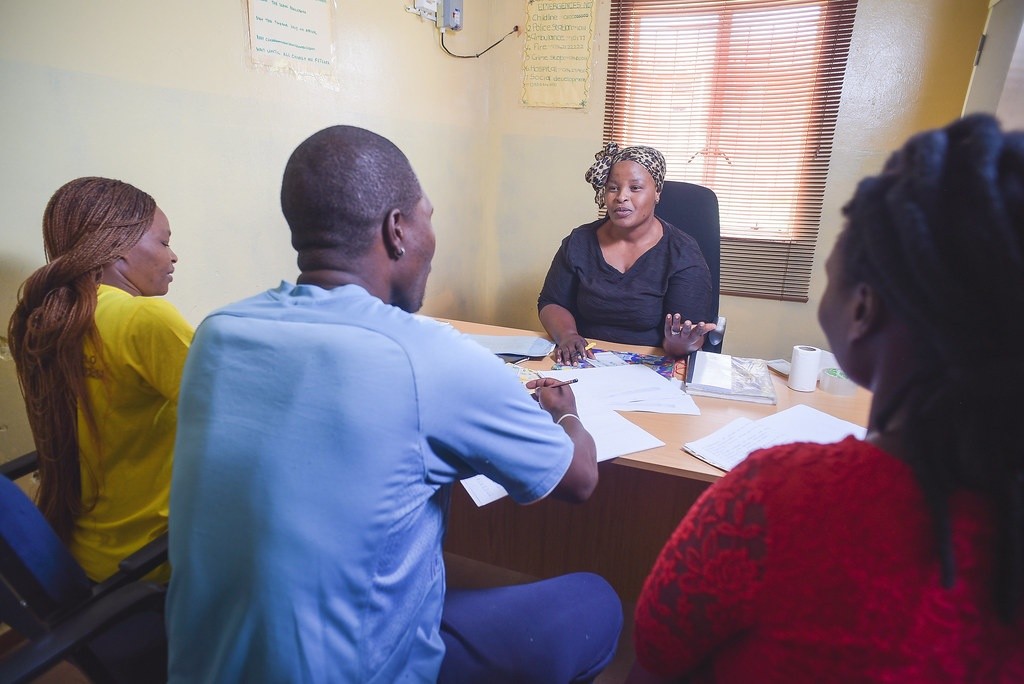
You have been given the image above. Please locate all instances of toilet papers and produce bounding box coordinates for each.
[787,345,837,393]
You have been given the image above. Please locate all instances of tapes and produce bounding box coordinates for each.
[820,368,858,395]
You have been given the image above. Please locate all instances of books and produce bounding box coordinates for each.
[685,350,777,406]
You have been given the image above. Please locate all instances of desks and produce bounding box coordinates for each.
[419,311,871,684]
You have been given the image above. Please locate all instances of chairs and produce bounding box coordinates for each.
[652,179,728,353]
[0,449,170,684]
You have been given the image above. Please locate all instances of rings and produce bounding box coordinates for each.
[671,326,683,335]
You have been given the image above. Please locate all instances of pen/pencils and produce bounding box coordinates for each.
[571,343,596,358]
[547,378,578,388]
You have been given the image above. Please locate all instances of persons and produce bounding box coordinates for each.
[6,177,195,593]
[632,112,1024,684]
[537,140,717,366]
[165,124,625,684]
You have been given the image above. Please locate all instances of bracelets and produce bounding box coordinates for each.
[557,414,582,424]
